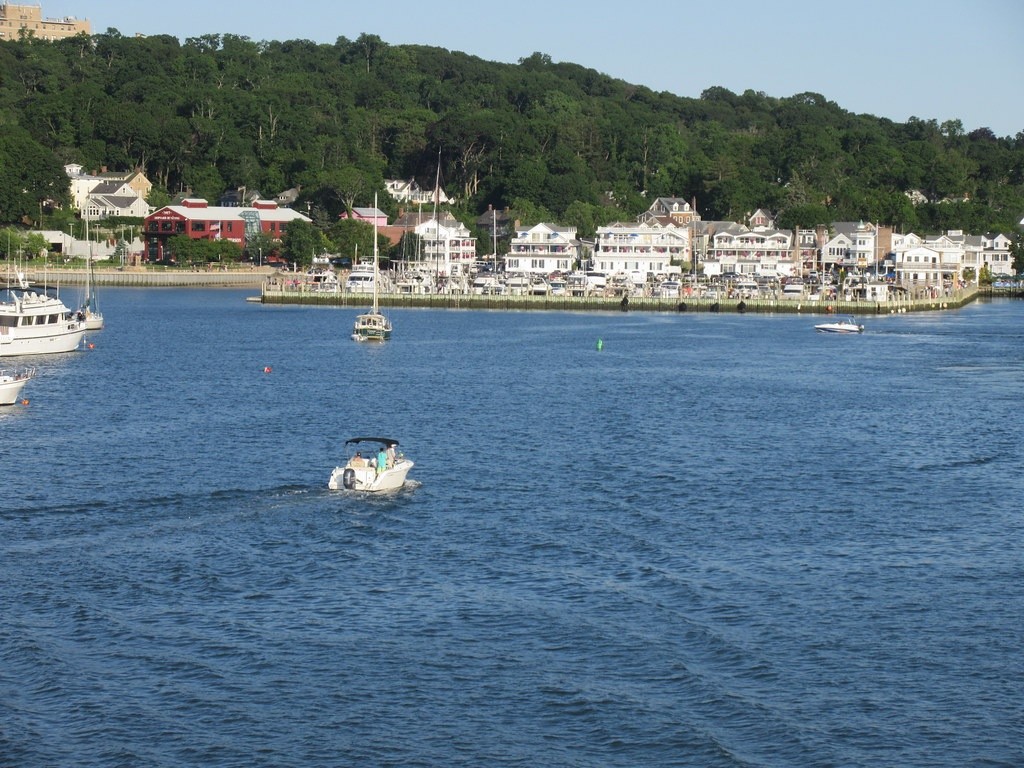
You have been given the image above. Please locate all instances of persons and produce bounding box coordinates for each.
[376,447,388,476]
[66,312,86,329]
[366,443,398,469]
[353,452,362,461]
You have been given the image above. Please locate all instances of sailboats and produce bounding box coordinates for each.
[74,198,104,331]
[350,191,394,344]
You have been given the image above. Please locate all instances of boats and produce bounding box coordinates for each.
[0,367,37,406]
[0,230,89,357]
[327,436,415,492]
[814,315,865,334]
[265,146,1024,300]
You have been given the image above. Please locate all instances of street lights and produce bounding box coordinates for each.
[120,223,126,243]
[121,248,124,267]
[129,225,136,255]
[94,223,101,251]
[68,222,75,256]
[160,245,163,260]
[259,248,263,266]
